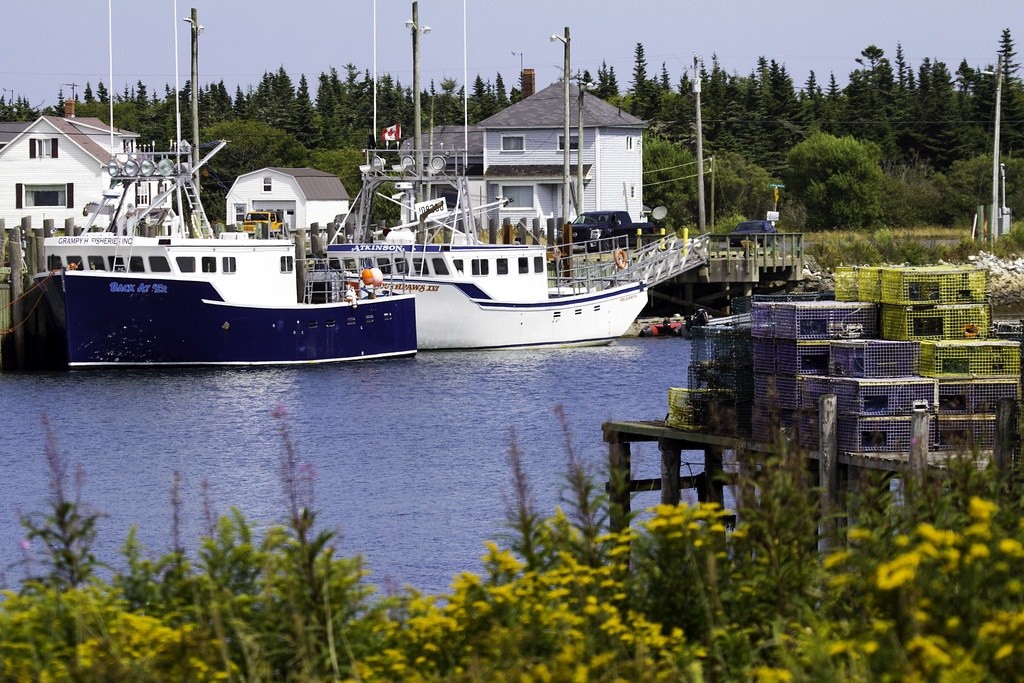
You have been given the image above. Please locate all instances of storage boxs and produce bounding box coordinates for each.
[665,267,1024,454]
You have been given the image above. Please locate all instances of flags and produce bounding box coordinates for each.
[382,123,401,141]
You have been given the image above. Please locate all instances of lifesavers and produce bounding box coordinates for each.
[615,248,626,270]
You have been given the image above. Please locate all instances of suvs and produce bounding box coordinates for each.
[241,209,287,236]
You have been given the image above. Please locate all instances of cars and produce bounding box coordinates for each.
[727,220,778,246]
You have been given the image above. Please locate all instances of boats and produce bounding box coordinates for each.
[306,0,649,355]
[640,319,687,337]
[28,0,418,370]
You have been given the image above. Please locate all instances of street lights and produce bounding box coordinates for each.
[549,33,570,223]
[981,71,1002,247]
[578,82,594,224]
[1000,163,1008,216]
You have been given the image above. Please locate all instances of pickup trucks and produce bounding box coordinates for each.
[558,211,654,254]
